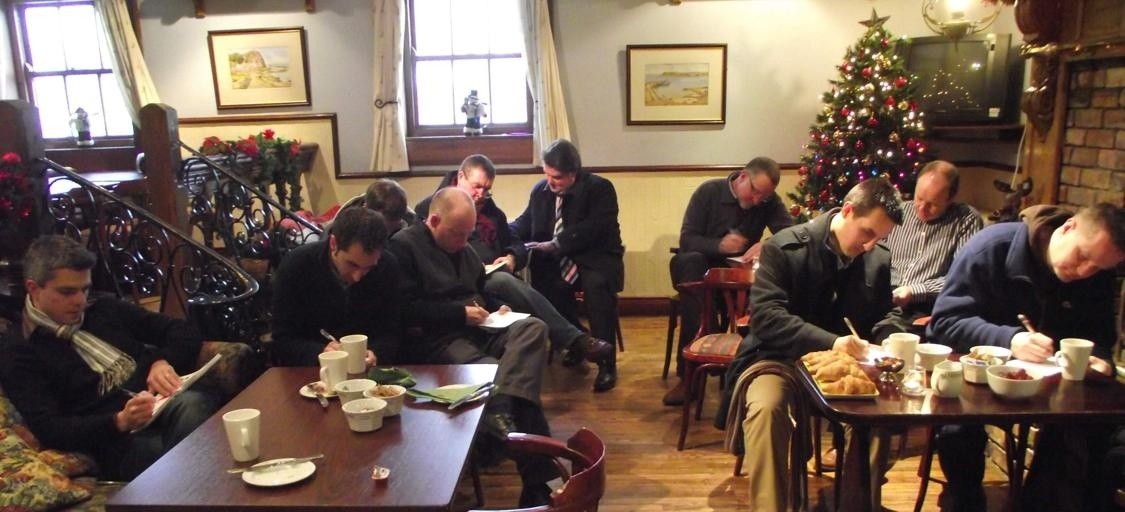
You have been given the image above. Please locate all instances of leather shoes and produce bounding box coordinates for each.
[560,330,614,367]
[478,412,518,442]
[814,447,837,472]
[662,376,699,405]
[594,364,617,390]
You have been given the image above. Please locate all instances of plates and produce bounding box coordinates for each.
[299,382,337,398]
[241,457,316,487]
[804,358,881,400]
[433,383,489,404]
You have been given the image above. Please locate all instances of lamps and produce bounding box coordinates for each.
[921,0,1003,37]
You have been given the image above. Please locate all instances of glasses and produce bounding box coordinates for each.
[748,173,774,204]
[462,171,493,200]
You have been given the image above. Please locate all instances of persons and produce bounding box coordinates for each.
[383,188,558,507]
[507,138,624,391]
[1,234,218,482]
[662,156,794,405]
[712,177,908,512]
[925,201,1125,512]
[272,155,615,366]
[813,157,984,472]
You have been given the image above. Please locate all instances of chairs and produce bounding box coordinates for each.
[468,427,606,512]
[677,267,757,451]
[548,245,627,366]
[661,247,683,380]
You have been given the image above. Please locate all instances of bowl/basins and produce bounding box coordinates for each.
[917,345,1044,399]
[335,379,408,433]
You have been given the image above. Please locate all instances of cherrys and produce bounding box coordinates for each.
[995,368,1034,381]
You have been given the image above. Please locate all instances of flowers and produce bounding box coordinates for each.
[197,128,302,184]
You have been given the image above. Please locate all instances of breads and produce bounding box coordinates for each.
[801,347,876,397]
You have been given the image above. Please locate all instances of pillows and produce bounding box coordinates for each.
[10,422,96,478]
[0,426,91,512]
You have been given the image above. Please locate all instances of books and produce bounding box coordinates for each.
[129,353,222,433]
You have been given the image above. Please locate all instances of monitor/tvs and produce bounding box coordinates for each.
[895,32,1024,125]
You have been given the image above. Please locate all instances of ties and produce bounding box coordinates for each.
[553,196,580,285]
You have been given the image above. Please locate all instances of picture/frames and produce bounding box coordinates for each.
[626,43,728,126]
[207,26,311,110]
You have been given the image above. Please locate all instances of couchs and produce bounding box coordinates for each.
[0,340,252,512]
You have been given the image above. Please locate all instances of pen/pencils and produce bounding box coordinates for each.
[473,300,479,307]
[121,388,136,397]
[1017,314,1035,332]
[844,317,860,340]
[320,328,337,341]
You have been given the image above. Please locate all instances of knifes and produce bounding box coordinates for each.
[308,383,329,409]
[447,383,495,412]
[226,453,326,473]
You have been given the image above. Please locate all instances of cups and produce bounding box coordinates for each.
[222,408,261,462]
[339,334,368,375]
[881,332,921,375]
[319,350,349,395]
[1054,338,1094,381]
